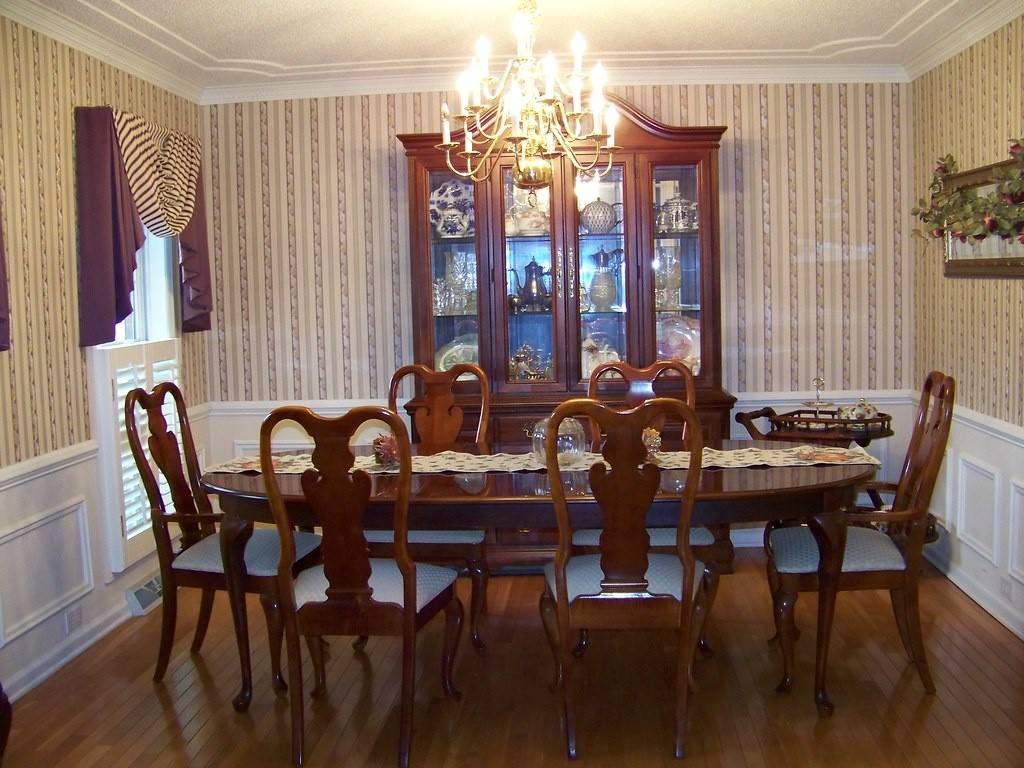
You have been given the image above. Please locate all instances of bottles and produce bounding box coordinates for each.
[544,353,554,381]
[535,349,543,363]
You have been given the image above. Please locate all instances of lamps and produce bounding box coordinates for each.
[435,0,623,210]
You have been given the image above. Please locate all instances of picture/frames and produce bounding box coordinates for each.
[937,157,1024,280]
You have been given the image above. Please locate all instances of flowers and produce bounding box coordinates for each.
[374,433,401,466]
[909,137,1024,246]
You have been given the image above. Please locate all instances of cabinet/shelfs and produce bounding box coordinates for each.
[395,90,736,574]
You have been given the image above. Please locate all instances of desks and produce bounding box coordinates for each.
[197,438,879,721]
[735,406,940,576]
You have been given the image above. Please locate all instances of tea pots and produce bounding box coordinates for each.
[505,214,520,236]
[848,398,877,426]
[509,256,552,313]
[580,334,610,380]
[577,197,624,235]
[585,244,625,311]
[654,192,698,231]
[516,208,547,236]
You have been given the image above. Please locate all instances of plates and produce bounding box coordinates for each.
[656,315,695,361]
[440,345,479,381]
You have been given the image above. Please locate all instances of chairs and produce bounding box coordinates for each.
[125,382,329,698]
[765,371,956,700]
[538,398,719,760]
[259,405,464,768]
[571,360,719,659]
[351,363,491,656]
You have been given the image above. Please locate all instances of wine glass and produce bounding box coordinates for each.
[443,251,467,314]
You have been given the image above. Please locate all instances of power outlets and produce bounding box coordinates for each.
[65,605,82,635]
